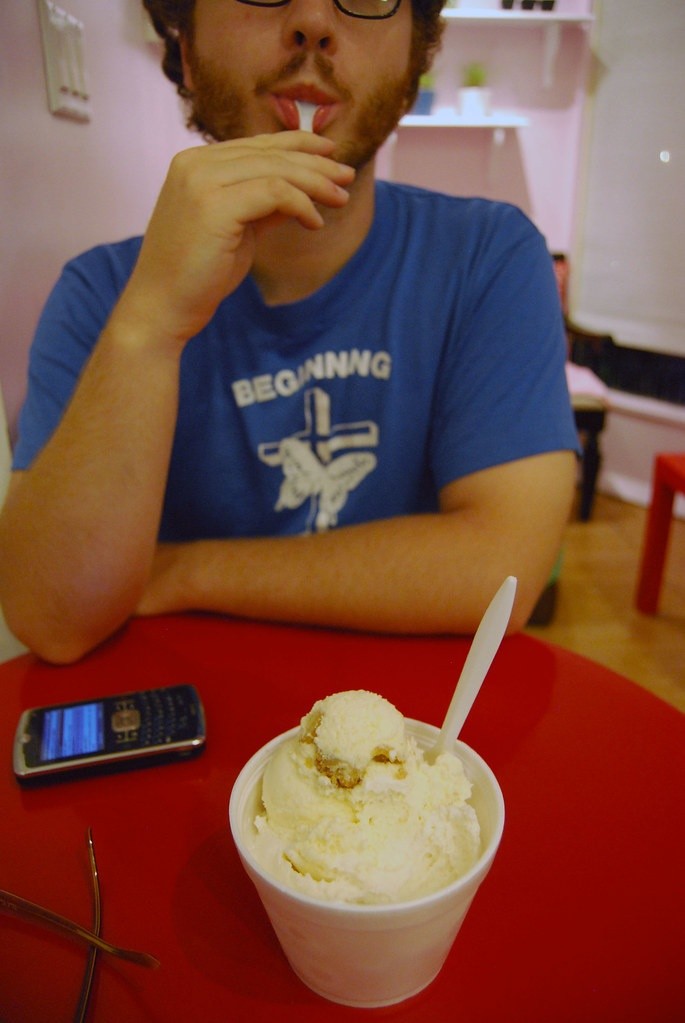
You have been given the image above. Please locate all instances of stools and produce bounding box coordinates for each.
[632,446,685,617]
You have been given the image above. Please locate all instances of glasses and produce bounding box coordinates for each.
[239,0,403,19]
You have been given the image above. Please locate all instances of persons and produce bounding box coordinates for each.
[0,0,585,666]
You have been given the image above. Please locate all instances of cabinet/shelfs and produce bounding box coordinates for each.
[384,4,596,162]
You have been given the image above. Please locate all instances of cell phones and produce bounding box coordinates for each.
[11,685,206,786]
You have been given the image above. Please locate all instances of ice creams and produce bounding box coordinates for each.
[247,688,481,905]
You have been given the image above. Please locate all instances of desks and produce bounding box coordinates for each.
[0,607,685,1023]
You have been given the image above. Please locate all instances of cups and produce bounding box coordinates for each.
[228,718,505,1007]
